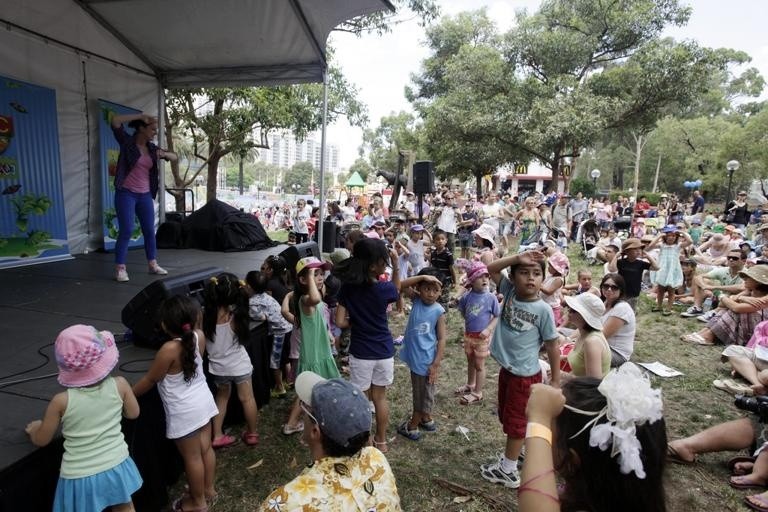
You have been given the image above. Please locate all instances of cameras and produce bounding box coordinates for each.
[733,394,768,426]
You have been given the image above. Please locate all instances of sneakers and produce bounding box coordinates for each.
[498,449,525,466]
[112,269,129,282]
[479,462,521,490]
[172,378,306,511]
[148,264,168,274]
[652,305,716,323]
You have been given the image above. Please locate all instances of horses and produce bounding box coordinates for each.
[376,169,408,191]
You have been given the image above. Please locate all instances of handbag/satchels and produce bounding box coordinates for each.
[721,211,734,223]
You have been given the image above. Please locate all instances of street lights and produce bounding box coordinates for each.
[723,158,739,221]
[590,169,600,203]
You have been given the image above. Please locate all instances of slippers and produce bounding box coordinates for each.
[667,334,767,511]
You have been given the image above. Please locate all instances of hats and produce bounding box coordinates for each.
[294,370,371,447]
[662,218,768,287]
[406,192,415,196]
[54,325,120,388]
[622,238,646,254]
[564,292,607,331]
[296,223,570,289]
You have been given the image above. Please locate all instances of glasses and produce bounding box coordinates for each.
[602,284,619,291]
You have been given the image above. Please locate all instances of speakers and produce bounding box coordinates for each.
[279,241,321,285]
[314,220,336,253]
[120,265,223,348]
[413,159,437,194]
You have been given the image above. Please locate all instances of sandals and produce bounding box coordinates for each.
[370,384,483,456]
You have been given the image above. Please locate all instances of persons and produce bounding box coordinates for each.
[132,292,219,511]
[200,273,259,448]
[25,324,140,512]
[231,181,768,512]
[111,113,177,283]
